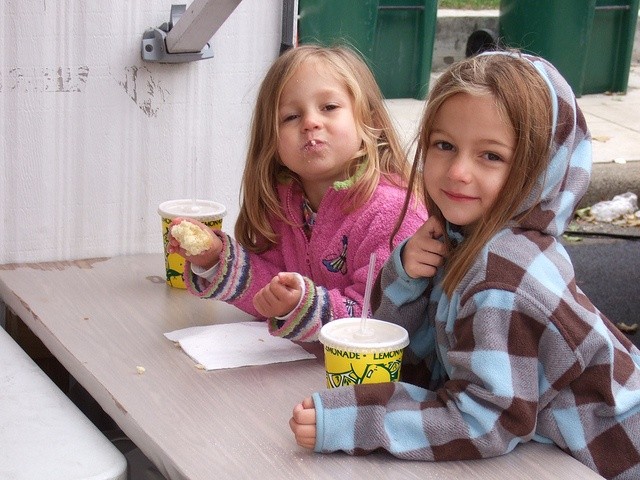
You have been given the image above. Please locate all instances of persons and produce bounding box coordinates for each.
[289,50,640,480]
[167,41,428,344]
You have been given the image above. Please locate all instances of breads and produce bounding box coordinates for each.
[171,219,213,256]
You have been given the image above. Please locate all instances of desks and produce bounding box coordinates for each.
[1,251,609,479]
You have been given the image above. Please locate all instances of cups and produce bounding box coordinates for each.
[317,318,410,390]
[158,198,228,289]
